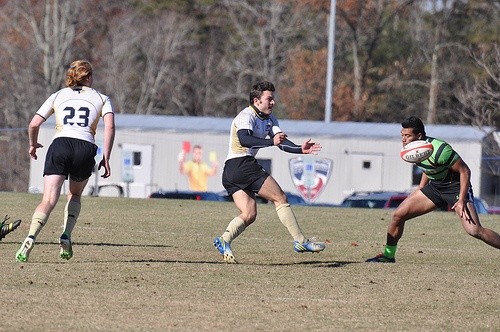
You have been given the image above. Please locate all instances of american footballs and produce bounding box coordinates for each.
[400,140,434,163]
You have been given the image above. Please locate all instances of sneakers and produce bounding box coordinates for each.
[213,236,238,264]
[59,231,73,260]
[0,215,21,241]
[293,238,325,253]
[365,252,395,263]
[16,235,35,262]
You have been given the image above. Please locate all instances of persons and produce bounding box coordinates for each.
[179,140,218,192]
[213,80,325,266]
[15,60,115,265]
[364,116,500,262]
